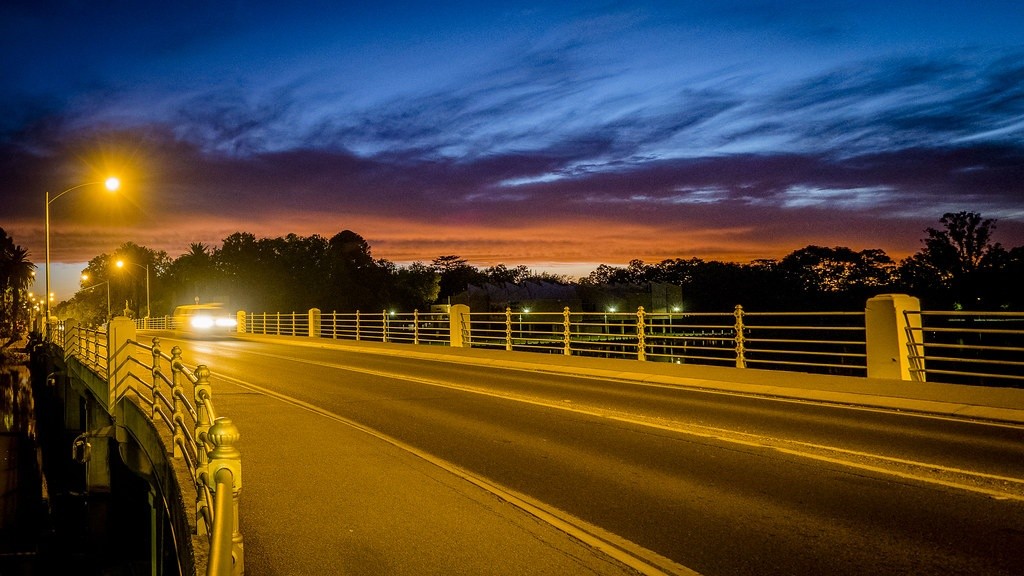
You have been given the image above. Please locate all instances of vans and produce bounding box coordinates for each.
[171,305,229,339]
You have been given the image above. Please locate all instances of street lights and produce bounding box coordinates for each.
[116,260,150,320]
[388,312,394,340]
[82,275,112,321]
[669,307,680,334]
[520,310,529,343]
[45,178,119,343]
[605,307,615,332]
